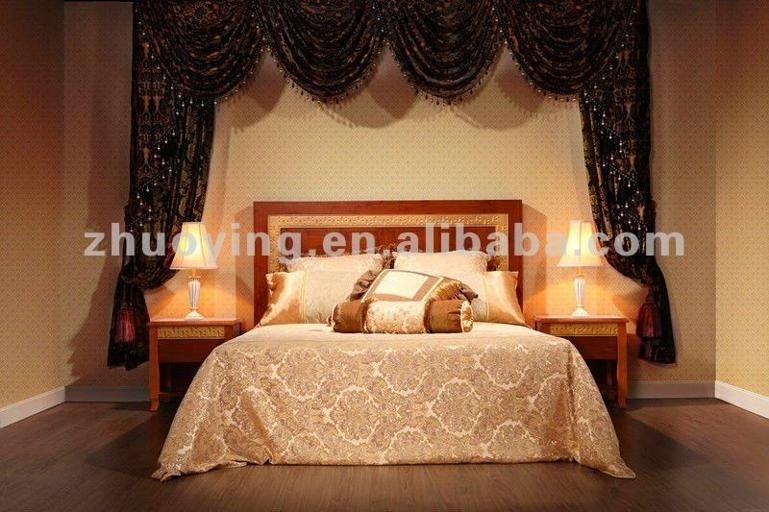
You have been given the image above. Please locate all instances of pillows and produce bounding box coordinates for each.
[256,248,526,333]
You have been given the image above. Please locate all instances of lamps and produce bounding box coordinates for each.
[557,219,603,317]
[169,222,218,321]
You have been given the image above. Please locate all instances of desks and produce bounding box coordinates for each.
[146,319,242,411]
[533,315,630,410]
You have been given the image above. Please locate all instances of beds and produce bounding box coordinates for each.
[212,200,569,466]
